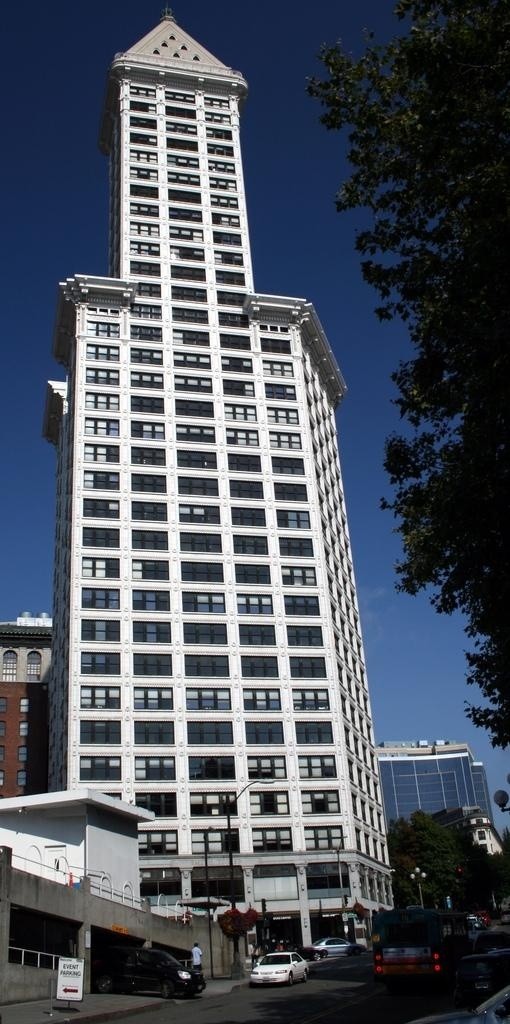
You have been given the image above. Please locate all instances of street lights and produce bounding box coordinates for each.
[337,834,350,942]
[493,773,510,814]
[225,776,276,979]
[409,867,427,909]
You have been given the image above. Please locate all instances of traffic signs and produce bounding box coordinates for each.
[55,957,83,1000]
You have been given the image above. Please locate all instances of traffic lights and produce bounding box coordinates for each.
[343,895,348,904]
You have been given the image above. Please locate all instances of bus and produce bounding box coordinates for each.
[370,903,474,981]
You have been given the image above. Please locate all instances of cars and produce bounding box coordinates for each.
[276,938,326,962]
[250,952,310,986]
[313,936,367,957]
[487,947,510,961]
[405,984,509,1023]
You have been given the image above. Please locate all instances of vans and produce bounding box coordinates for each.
[93,946,205,999]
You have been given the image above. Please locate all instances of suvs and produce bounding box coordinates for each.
[475,910,490,927]
[475,931,509,955]
[454,952,509,1006]
[500,909,510,924]
[464,912,484,930]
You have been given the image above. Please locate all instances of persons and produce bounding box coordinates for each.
[256,939,304,957]
[191,941,204,975]
[247,941,258,972]
